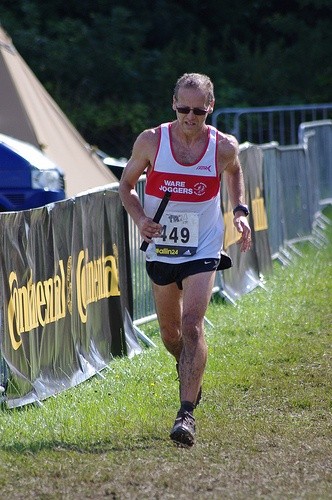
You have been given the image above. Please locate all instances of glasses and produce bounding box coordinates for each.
[175,105,210,116]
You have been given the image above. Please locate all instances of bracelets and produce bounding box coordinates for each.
[233,205,251,218]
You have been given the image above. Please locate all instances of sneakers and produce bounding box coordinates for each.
[168,408,200,449]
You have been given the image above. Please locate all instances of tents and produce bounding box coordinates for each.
[1,30,121,199]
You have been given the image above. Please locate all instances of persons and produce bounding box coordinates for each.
[120,75,253,447]
